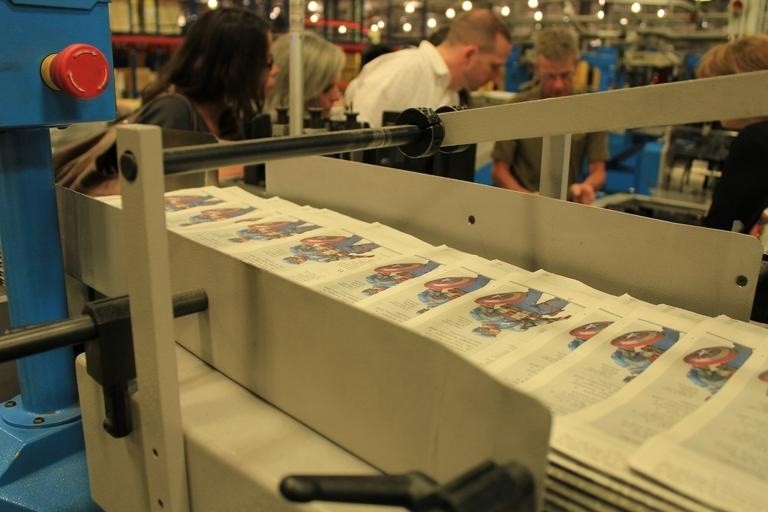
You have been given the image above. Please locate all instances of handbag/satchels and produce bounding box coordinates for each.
[49,121,116,190]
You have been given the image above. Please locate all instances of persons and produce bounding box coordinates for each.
[359,41,401,69]
[346,10,512,130]
[490,26,608,206]
[609,60,683,173]
[429,24,476,173]
[260,29,347,121]
[52,7,279,197]
[698,35,768,226]
